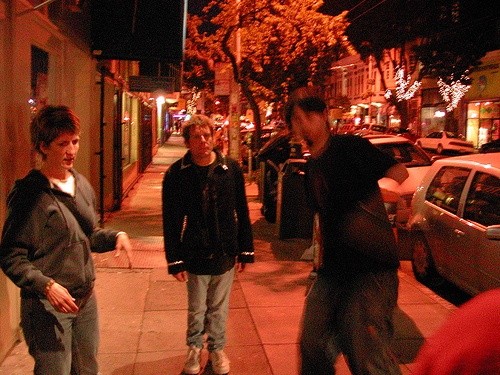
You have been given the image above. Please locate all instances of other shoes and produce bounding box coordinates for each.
[208,350,230,374]
[182,346,201,375]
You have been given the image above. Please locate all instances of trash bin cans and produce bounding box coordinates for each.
[254,135,311,240]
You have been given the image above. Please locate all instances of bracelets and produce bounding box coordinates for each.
[42,280,54,298]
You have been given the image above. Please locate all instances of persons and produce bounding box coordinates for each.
[161,116,254,374]
[0,105,135,375]
[284,90,409,375]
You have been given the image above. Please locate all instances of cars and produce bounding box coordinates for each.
[333,123,420,141]
[415,130,480,154]
[406,152,500,298]
[360,135,433,225]
